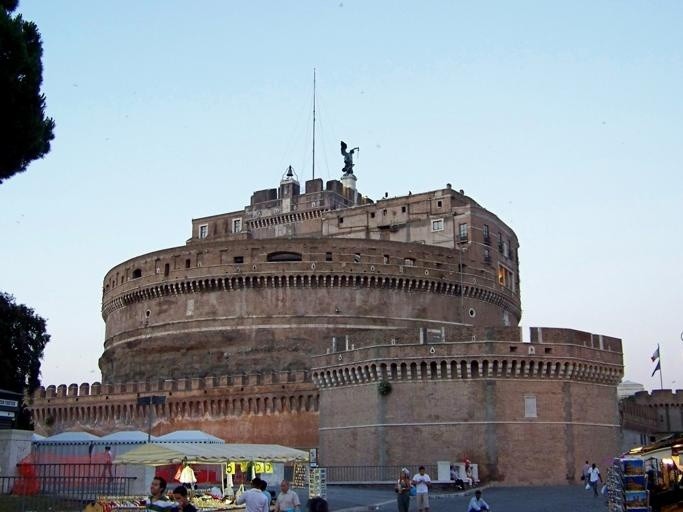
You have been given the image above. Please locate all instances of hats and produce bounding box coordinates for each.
[402,467,410,477]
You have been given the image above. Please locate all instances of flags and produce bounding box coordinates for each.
[650,347,659,362]
[650,360,660,378]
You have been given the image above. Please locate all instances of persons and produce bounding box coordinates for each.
[273,479,301,512]
[169,484,198,511]
[582,460,591,486]
[450,463,465,491]
[342,149,354,176]
[410,464,431,512]
[259,479,271,511]
[235,477,269,511]
[136,476,179,511]
[464,459,478,488]
[396,467,413,512]
[305,496,328,511]
[466,489,490,511]
[96,445,114,481]
[587,462,603,496]
